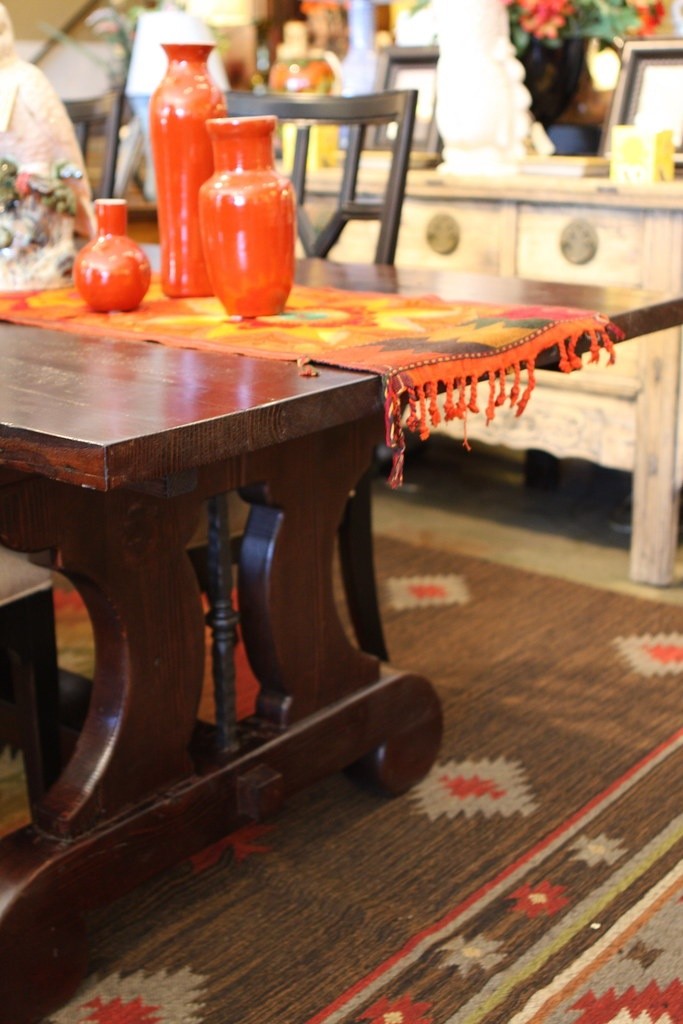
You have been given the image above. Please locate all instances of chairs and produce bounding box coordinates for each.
[158,90,418,664]
[0,545,70,826]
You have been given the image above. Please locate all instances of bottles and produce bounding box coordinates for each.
[268,21,339,169]
[75,197,150,311]
[149,41,227,298]
[198,115,300,316]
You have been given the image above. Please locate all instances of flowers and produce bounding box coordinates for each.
[402,0,665,57]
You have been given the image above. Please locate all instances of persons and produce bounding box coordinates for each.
[0,4,92,201]
[127,0,231,202]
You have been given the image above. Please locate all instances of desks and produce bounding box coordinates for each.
[0,236,683,1024]
[280,174,683,586]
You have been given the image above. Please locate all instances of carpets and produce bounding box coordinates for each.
[0,524,683,1024]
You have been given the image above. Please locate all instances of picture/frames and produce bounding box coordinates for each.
[364,43,443,154]
[599,34,683,167]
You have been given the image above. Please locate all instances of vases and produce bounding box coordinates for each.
[512,38,582,130]
[196,114,296,319]
[148,42,229,301]
[74,198,151,314]
